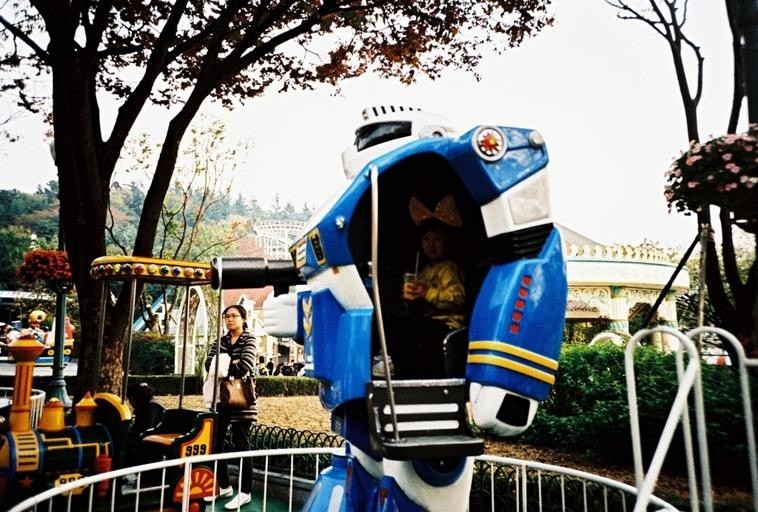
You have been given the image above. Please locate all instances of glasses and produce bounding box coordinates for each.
[224,314,244,319]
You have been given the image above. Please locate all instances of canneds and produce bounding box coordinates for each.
[404,272,417,300]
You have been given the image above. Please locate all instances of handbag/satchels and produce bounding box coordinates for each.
[220,360,256,410]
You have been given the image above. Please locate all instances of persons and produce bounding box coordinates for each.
[254,354,306,377]
[119,381,165,486]
[370,194,472,380]
[205,304,258,512]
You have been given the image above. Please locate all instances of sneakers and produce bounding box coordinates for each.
[224,492,252,510]
[371,353,397,378]
[202,484,235,502]
